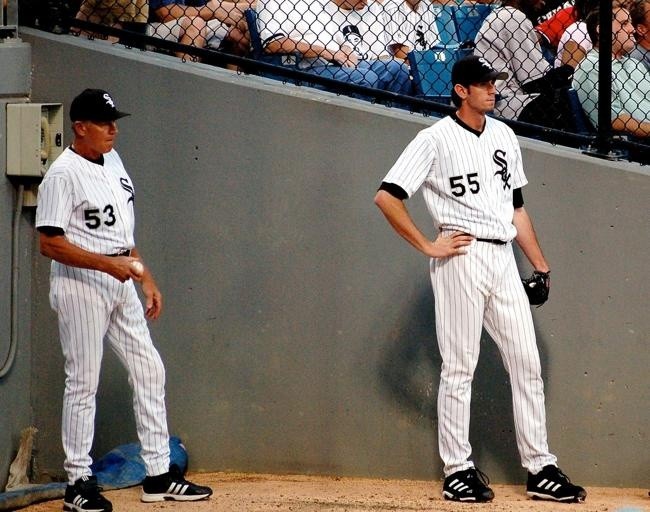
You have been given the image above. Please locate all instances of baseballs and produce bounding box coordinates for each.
[129,261,144,274]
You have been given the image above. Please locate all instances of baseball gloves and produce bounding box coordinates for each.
[521,270,549,305]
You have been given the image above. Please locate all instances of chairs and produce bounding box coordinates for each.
[409,51,463,99]
[245,7,302,83]
[565,89,636,157]
[430,3,491,46]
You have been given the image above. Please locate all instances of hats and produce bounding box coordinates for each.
[70,88,130,124]
[343,25,360,39]
[451,56,508,85]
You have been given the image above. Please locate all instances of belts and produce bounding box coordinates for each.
[477,238,507,245]
[105,250,130,257]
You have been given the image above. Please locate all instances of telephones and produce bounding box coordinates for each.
[41,108,51,163]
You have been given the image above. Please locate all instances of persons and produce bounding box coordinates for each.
[374,56,586,502]
[35,88,213,512]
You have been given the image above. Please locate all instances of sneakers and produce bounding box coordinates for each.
[443,468,494,501]
[141,465,212,503]
[64,475,112,512]
[526,464,586,502]
